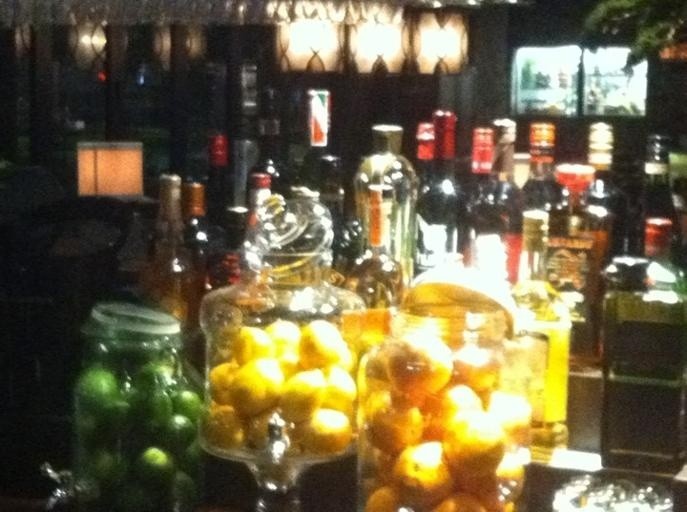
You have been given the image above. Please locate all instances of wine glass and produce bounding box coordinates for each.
[1,0,403,31]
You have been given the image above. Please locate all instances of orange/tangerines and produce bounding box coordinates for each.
[72,360,206,511]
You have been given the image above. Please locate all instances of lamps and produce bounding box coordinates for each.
[74,139,146,203]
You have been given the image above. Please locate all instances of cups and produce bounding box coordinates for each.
[552,471,674,511]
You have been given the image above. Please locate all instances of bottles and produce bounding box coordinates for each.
[546,159,612,306]
[64,104,571,512]
[579,120,629,258]
[626,214,686,297]
[620,129,687,266]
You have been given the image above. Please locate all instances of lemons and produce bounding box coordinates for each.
[209,318,359,457]
[358,306,532,511]
